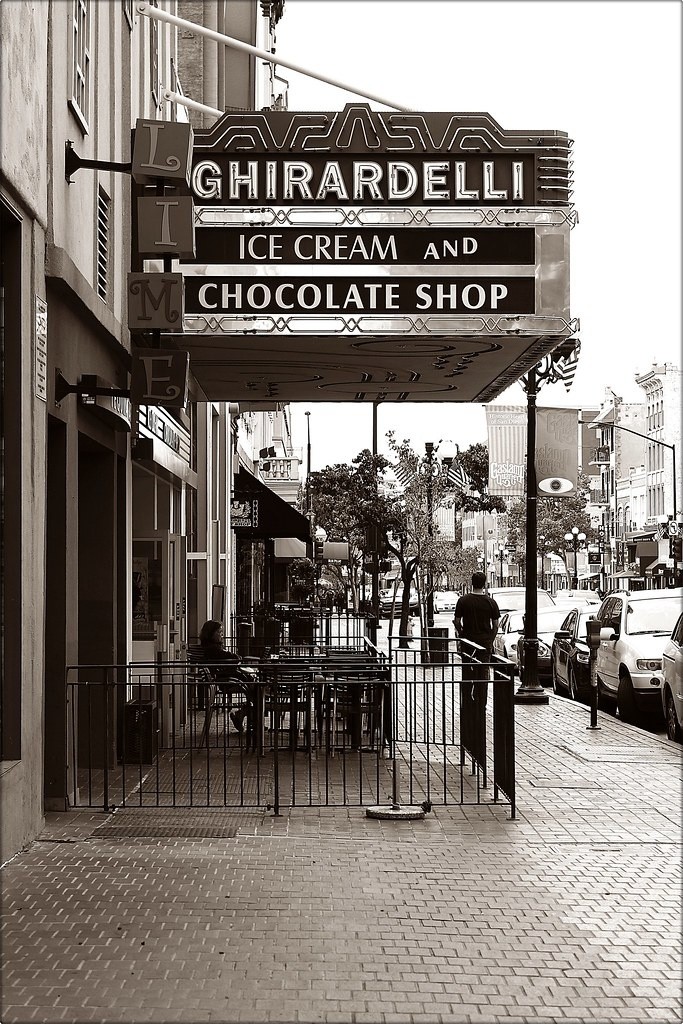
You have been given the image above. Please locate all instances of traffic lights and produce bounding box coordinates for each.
[672,538,682,561]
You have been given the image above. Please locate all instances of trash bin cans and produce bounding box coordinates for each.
[426,627,449,663]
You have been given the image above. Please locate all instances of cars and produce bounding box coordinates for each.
[432,591,459,613]
[482,587,682,744]
[359,576,421,619]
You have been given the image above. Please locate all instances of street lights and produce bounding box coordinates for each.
[477,552,492,575]
[578,419,677,574]
[537,535,553,589]
[564,526,587,589]
[494,544,510,587]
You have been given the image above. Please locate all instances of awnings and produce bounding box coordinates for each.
[644,559,666,577]
[578,573,599,579]
[606,570,640,577]
[234,465,311,540]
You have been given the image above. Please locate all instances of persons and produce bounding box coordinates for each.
[259,620,282,705]
[199,620,268,732]
[454,571,500,708]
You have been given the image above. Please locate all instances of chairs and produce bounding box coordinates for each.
[196,643,388,758]
